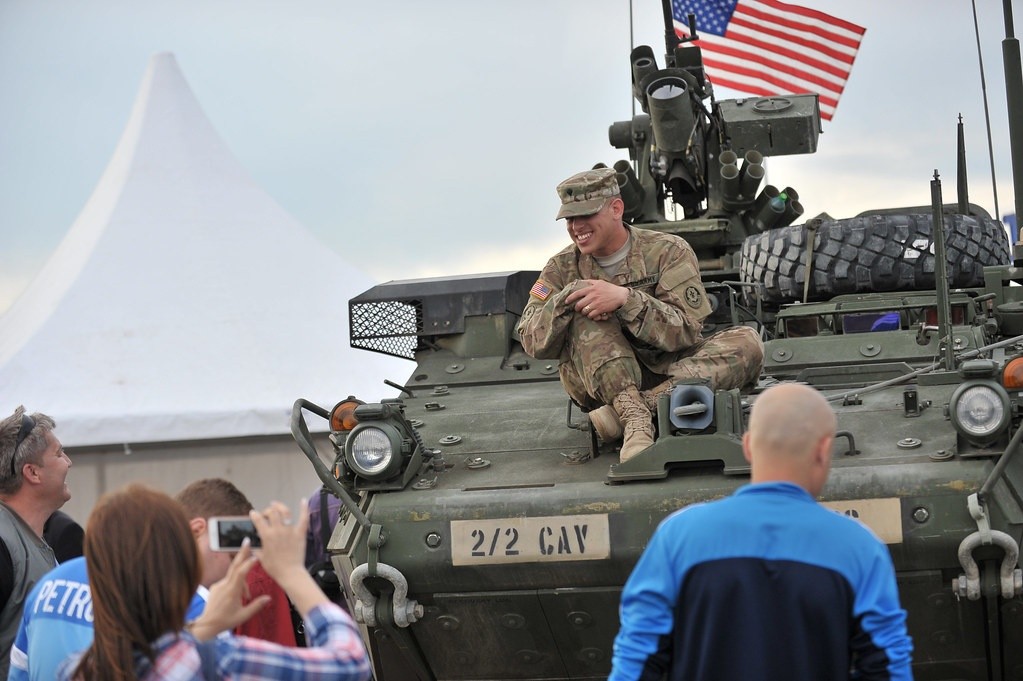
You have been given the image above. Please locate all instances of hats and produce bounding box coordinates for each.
[555,168,621,220]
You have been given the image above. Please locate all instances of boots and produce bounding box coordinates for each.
[588,379,676,442]
[614,385,656,463]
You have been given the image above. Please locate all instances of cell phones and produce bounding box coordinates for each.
[208,517,262,551]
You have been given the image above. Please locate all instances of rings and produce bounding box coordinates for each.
[282,519,294,526]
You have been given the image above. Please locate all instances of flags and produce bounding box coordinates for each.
[671,1,866,121]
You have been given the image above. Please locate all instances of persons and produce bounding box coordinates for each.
[234,558,304,647]
[612,382,916,681]
[518,169,767,463]
[69,484,372,681]
[308,487,342,554]
[8,478,255,681]
[0,406,88,681]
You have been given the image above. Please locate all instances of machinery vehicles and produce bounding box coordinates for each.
[290,0,1022,679]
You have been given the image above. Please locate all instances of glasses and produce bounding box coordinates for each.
[10,414,35,474]
